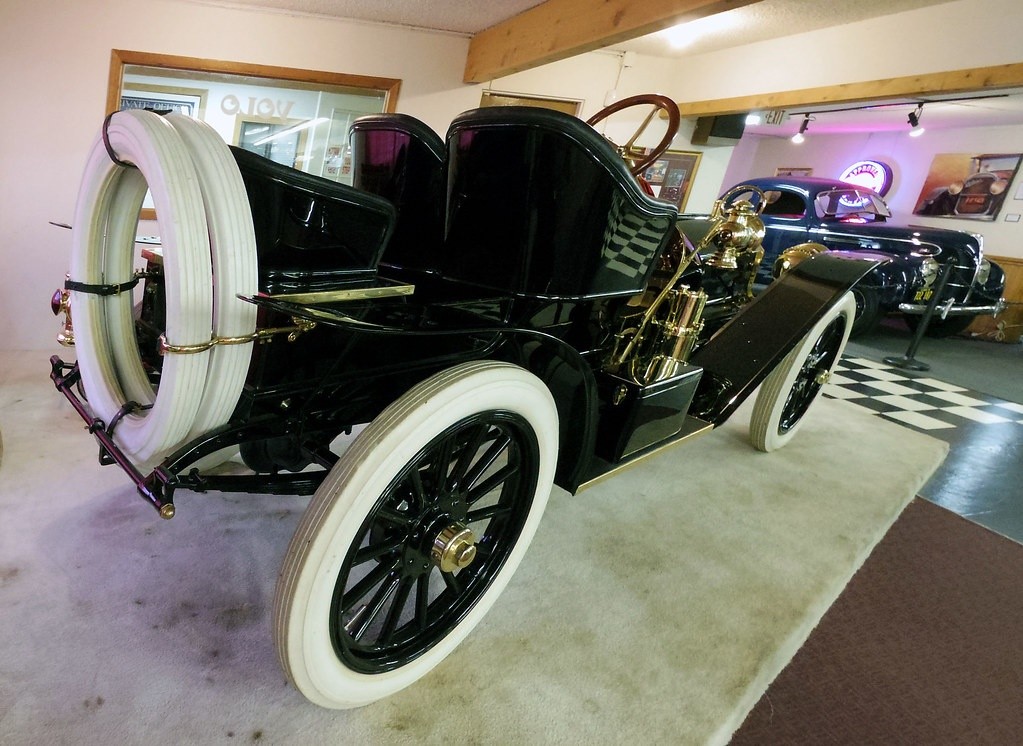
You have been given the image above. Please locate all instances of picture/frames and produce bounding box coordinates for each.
[774,168,813,177]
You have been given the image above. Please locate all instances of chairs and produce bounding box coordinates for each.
[348,113,447,279]
[443,106,681,300]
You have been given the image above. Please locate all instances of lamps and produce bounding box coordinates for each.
[907,102,925,136]
[792,113,817,143]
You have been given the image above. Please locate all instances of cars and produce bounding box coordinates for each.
[48,92,895,713]
[718,175,1009,343]
